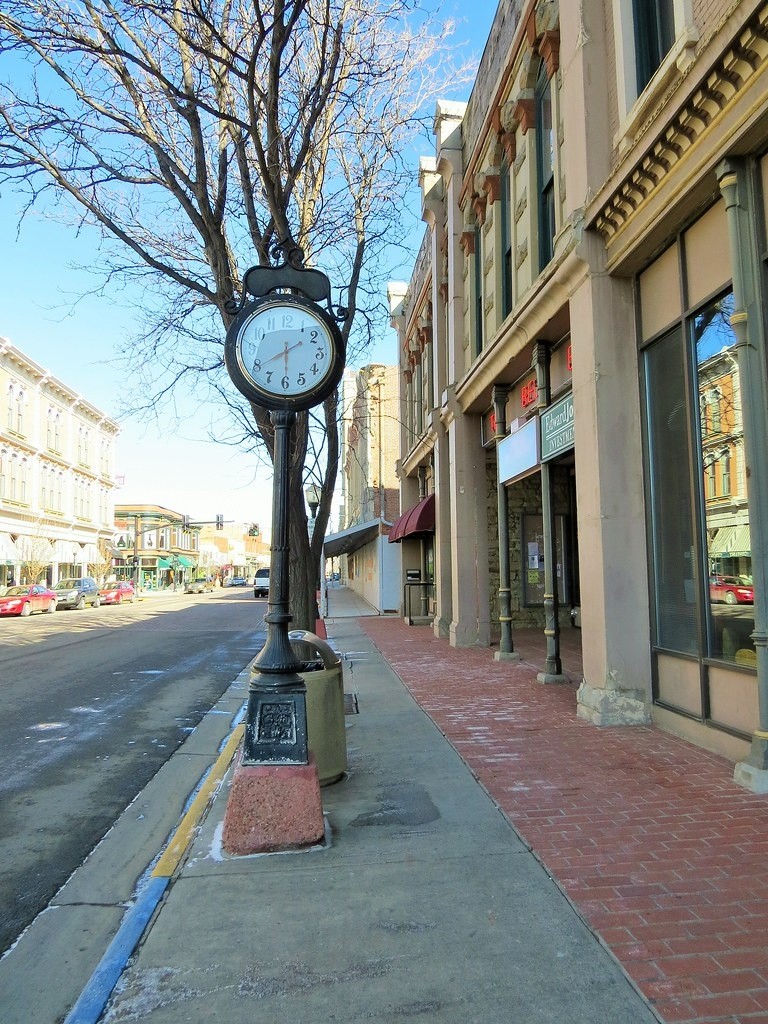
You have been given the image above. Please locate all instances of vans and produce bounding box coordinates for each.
[253,567,270,599]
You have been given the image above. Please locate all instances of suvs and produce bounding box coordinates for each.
[50,577,101,610]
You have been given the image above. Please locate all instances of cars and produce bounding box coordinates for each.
[231,577,247,587]
[98,581,135,605]
[188,577,214,593]
[0,584,58,617]
[709,576,756,606]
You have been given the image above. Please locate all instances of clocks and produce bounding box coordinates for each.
[224,294,347,410]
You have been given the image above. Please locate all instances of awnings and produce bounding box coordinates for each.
[159,555,195,570]
[388,493,435,543]
[320,518,380,615]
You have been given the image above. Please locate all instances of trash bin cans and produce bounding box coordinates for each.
[250,629,347,788]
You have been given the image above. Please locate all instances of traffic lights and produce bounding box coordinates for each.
[249,525,259,536]
